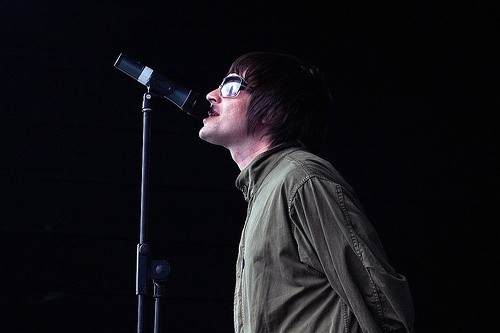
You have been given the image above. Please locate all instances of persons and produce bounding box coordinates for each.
[198,51,416,332]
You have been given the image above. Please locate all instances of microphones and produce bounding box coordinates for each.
[114,53,209,116]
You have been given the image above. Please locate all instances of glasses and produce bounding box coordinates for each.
[218,73,254,99]
